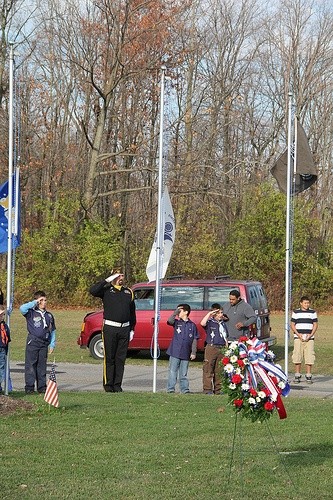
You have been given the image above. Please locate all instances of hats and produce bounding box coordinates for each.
[110,266,123,276]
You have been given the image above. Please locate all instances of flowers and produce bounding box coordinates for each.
[217,336,277,422]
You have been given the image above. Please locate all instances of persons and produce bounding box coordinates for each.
[223,290,257,339]
[166,304,200,394]
[201,303,229,395]
[20,290,56,395]
[0,305,13,395]
[88,266,136,392]
[290,297,318,384]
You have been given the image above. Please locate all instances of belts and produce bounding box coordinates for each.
[105,320,130,327]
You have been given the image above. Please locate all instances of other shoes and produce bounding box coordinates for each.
[184,390,190,394]
[104,386,122,393]
[40,390,45,393]
[167,388,174,392]
[26,389,36,394]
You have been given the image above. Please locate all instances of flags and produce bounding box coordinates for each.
[146,185,176,282]
[0,168,21,253]
[270,114,318,196]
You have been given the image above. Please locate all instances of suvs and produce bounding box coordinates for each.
[76,274,277,360]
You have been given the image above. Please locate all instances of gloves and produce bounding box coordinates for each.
[105,274,120,283]
[129,330,134,341]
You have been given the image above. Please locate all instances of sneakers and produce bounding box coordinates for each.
[293,374,302,384]
[305,375,312,383]
